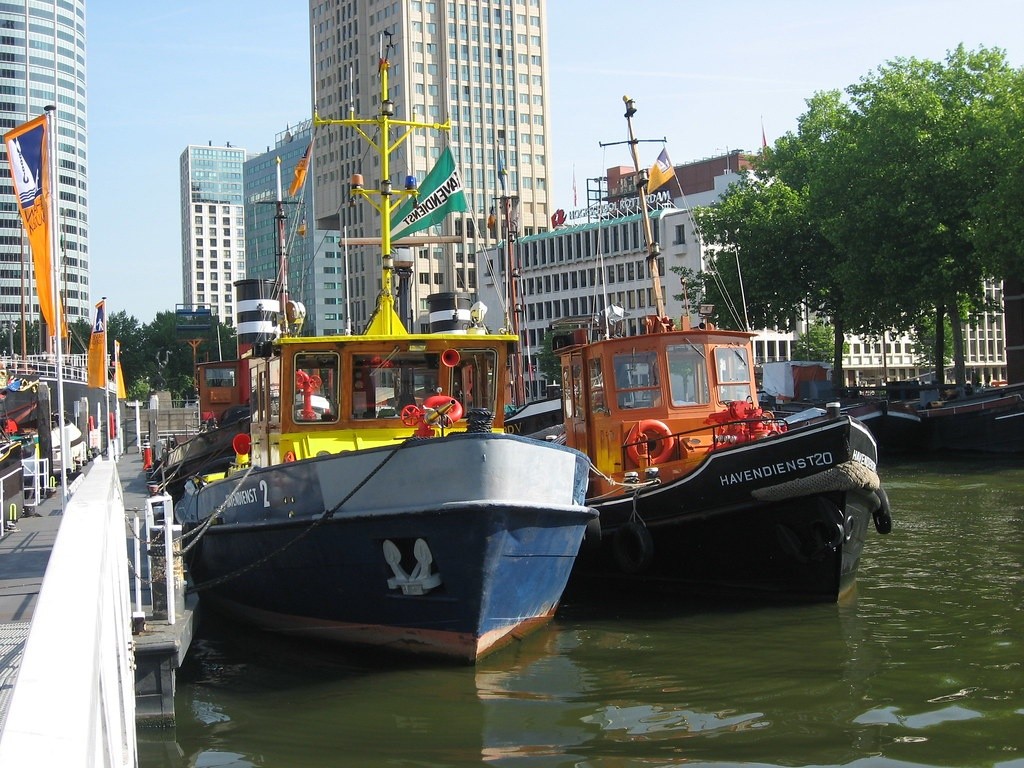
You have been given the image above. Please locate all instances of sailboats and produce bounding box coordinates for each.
[473,157,568,437]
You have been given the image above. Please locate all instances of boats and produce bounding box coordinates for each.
[175,31,591,669]
[147,404,252,484]
[0,397,88,483]
[0,350,126,437]
[754,380,1024,454]
[554,97,882,614]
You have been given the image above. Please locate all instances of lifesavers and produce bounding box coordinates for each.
[626,419,675,466]
[422,394,463,424]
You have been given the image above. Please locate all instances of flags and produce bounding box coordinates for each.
[648,148,675,195]
[390,146,470,242]
